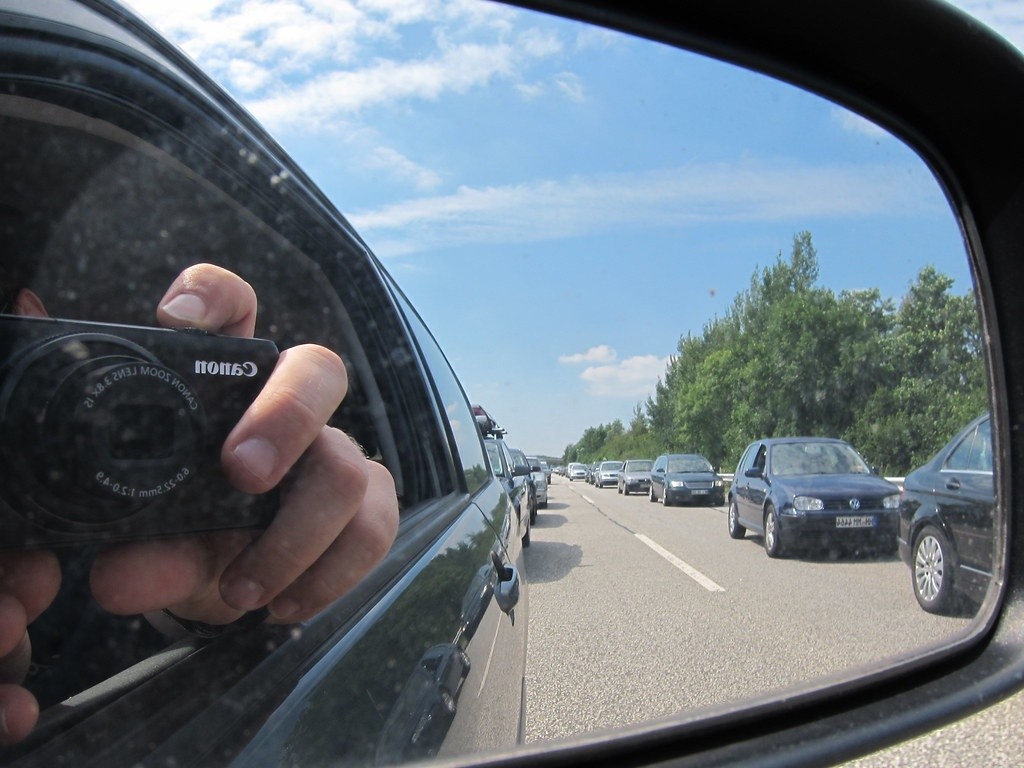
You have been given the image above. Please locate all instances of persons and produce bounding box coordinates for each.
[1,262,399,750]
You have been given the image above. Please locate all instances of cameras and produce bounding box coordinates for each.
[0,313,280,549]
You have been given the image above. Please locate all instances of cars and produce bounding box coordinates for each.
[618,459,655,495]
[649,454,725,507]
[469,403,602,550]
[595,460,625,488]
[728,437,903,560]
[892,406,998,617]
